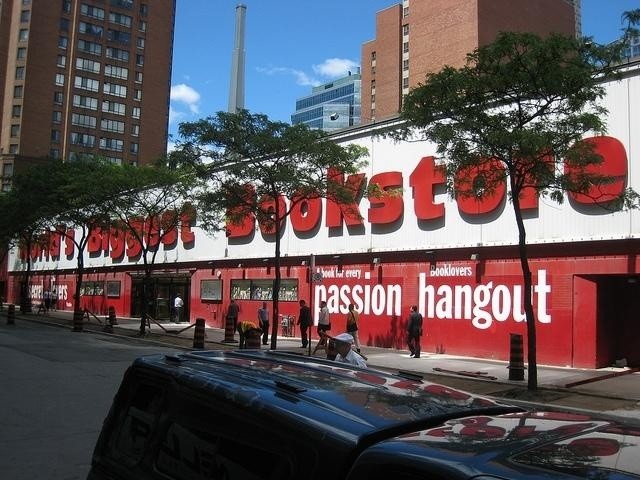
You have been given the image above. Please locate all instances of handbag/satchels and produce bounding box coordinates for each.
[346,310,358,332]
[415,312,423,336]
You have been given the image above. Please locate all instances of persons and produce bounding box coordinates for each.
[404,304,424,359]
[331,333,370,370]
[43,288,59,313]
[227,299,240,334]
[346,304,360,355]
[316,300,330,346]
[174,293,185,324]
[257,302,270,345]
[236,320,257,349]
[296,300,314,348]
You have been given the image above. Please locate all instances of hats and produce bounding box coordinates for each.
[332,333,355,345]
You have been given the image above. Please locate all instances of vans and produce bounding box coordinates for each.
[87,349,640,480]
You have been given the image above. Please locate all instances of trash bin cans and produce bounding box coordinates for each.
[59,301,65,310]
